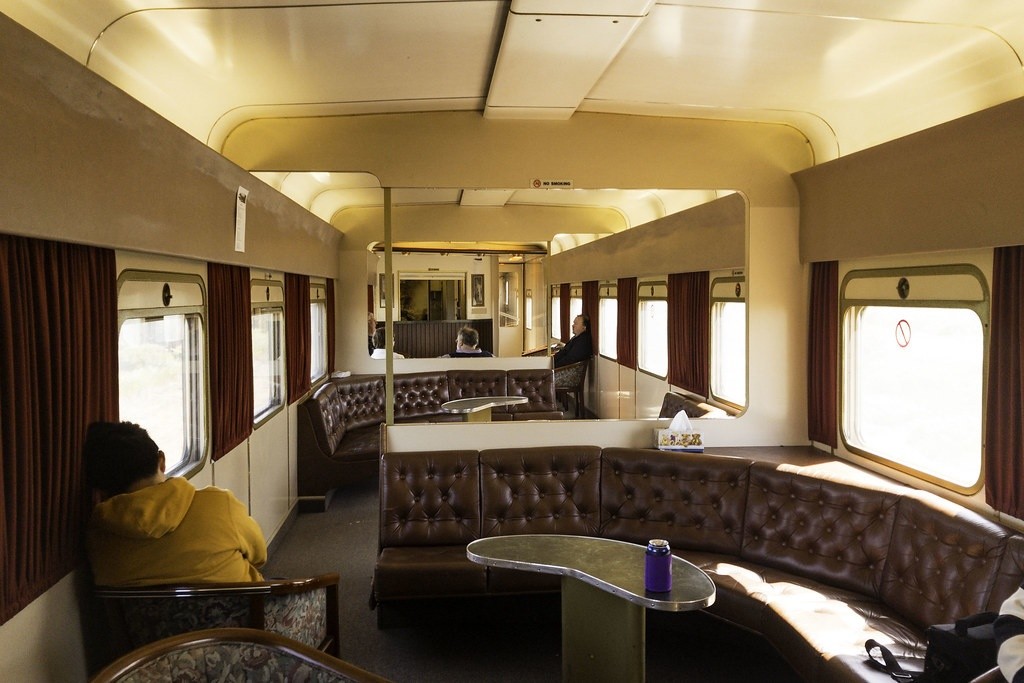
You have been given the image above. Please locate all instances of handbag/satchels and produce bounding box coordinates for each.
[922,609,1002,683]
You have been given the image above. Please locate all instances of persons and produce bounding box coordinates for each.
[79,418,287,590]
[371,327,406,358]
[547,314,593,369]
[439,326,496,357]
[367,311,379,356]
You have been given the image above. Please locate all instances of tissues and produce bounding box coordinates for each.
[651,409,705,453]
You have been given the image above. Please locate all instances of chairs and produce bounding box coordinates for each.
[75,554,393,683]
[521,344,589,419]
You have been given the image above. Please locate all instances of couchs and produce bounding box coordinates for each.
[294,366,1024,683]
